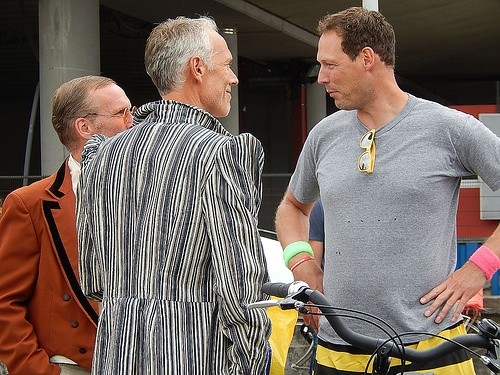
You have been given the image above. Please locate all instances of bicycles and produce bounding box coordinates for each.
[245,280,500,375]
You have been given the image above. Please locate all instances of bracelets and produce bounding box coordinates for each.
[469,246,500,280]
[283,242,313,271]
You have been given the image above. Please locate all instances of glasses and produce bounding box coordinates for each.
[358,129,375,173]
[81,106,137,124]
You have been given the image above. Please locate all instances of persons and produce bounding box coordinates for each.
[0,75,137,375]
[276,7,500,375]
[76,18,271,375]
